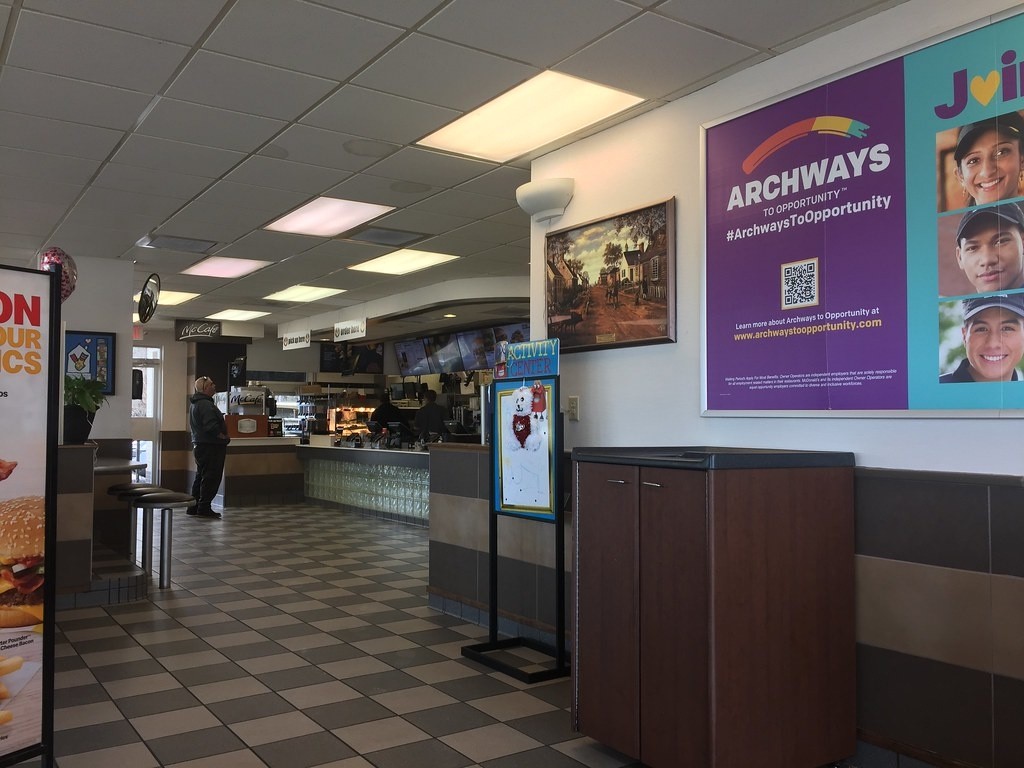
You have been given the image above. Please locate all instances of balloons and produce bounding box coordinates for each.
[40,247,77,305]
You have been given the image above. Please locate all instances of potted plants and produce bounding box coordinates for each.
[64,374,109,446]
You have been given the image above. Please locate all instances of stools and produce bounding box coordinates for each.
[106,483,156,564]
[118,487,175,576]
[133,492,197,590]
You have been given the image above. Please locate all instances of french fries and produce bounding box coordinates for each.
[0,656,22,724]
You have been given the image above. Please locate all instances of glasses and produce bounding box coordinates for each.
[203,376,208,390]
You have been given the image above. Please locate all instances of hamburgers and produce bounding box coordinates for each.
[495,328,508,342]
[0,497,45,627]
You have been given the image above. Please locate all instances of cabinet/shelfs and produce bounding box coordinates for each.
[568,445,857,768]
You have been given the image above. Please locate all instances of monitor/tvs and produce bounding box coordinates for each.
[387,422,411,435]
[443,421,468,434]
[404,382,417,399]
[365,421,382,433]
[390,382,405,400]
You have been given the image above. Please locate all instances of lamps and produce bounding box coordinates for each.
[515,177,574,220]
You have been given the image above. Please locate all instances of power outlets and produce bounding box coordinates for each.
[568,396,579,421]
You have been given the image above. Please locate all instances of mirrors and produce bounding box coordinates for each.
[138,273,161,323]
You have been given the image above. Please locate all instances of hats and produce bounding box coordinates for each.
[954,111,1024,160]
[956,203,1024,241]
[962,293,1024,325]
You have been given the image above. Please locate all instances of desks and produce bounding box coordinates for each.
[94,459,147,476]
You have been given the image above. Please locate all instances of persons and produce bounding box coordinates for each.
[952,110,1024,207]
[185,375,230,518]
[371,392,410,433]
[414,389,451,440]
[954,201,1024,295]
[938,291,1024,383]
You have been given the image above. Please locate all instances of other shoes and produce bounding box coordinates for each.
[198,505,221,518]
[187,503,198,515]
